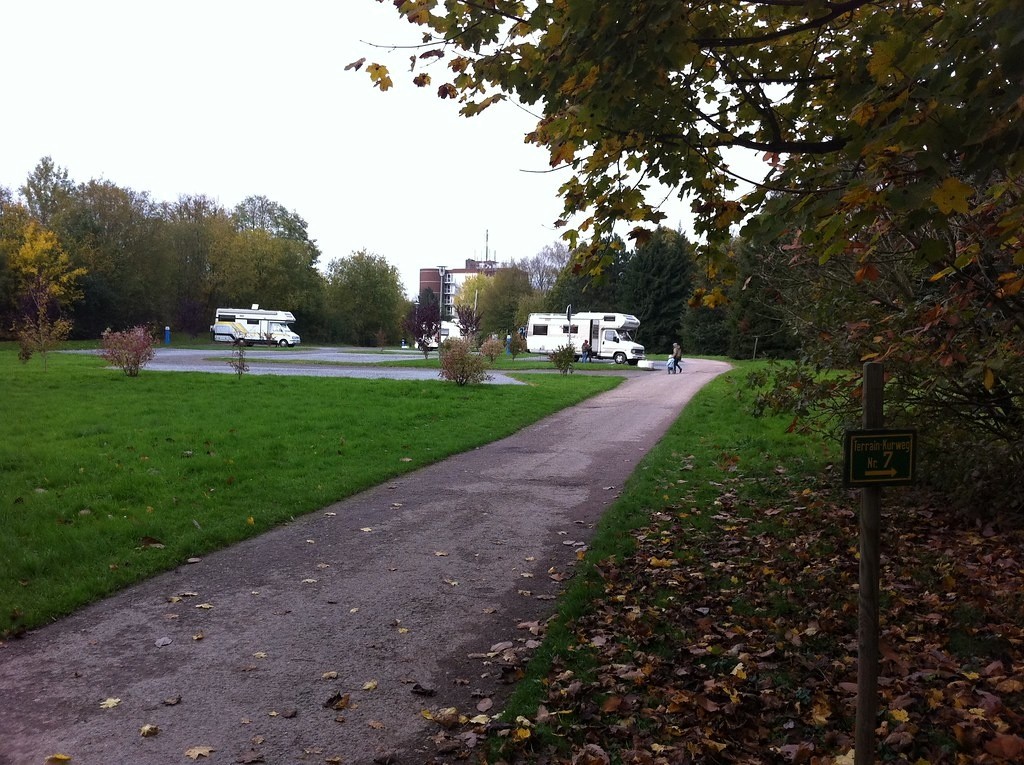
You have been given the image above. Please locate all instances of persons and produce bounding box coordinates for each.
[581,339,594,364]
[666,343,683,375]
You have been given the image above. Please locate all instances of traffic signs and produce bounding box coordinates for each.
[843,429,916,489]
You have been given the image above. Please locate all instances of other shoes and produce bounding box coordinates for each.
[680,369,682,372]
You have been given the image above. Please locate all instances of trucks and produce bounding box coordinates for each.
[209,304,301,348]
[414,318,472,351]
[527,311,646,365]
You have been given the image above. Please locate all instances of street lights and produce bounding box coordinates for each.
[436,265,447,350]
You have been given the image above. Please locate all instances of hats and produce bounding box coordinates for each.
[585,340,588,343]
[669,355,672,358]
